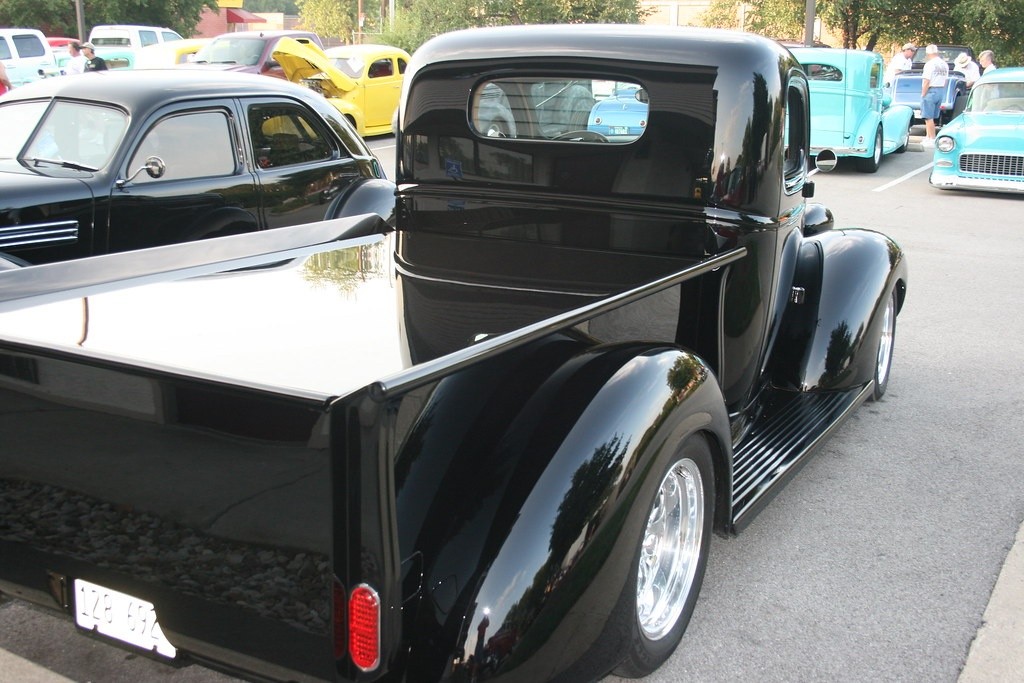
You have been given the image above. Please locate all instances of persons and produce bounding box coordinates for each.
[65,40,108,75]
[976,50,999,101]
[952,52,980,90]
[920,43,949,149]
[883,42,918,87]
[0,61,15,96]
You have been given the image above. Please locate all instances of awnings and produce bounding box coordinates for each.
[227,8,267,23]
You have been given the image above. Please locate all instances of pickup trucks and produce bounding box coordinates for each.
[0,28,134,89]
[0,24,909,683]
[182,29,324,82]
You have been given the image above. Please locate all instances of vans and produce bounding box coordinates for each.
[87,24,186,59]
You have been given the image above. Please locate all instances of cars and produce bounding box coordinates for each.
[471,79,596,139]
[273,36,410,142]
[890,42,984,119]
[0,65,397,269]
[786,48,915,172]
[927,66,1024,197]
[588,84,651,139]
[44,36,82,50]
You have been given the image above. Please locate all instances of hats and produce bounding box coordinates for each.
[954,53,972,68]
[78,42,95,51]
[926,44,938,54]
[902,42,917,53]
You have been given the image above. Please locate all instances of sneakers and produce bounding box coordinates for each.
[920,138,936,149]
